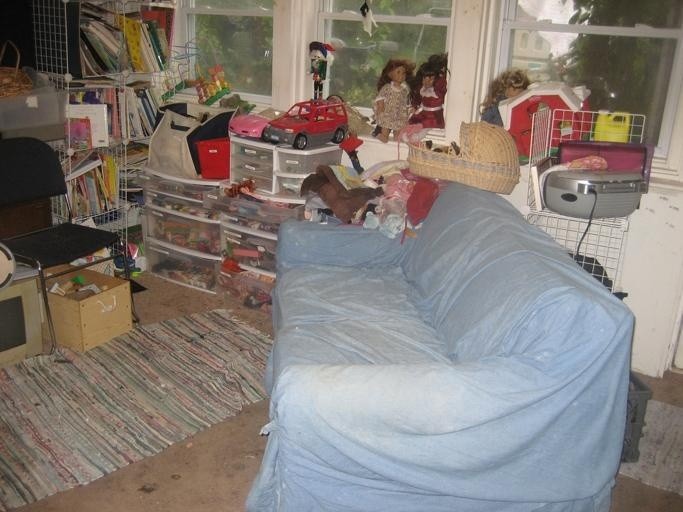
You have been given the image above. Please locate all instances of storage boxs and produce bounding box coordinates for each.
[142,133,342,309]
[1,264,134,370]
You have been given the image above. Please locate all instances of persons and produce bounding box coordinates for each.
[306,40,327,99]
[477,68,542,128]
[358,60,418,151]
[398,51,453,145]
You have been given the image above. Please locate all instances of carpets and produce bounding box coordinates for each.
[618,398,683,497]
[2,309,277,509]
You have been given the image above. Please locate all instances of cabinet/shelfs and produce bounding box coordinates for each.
[32,1,177,270]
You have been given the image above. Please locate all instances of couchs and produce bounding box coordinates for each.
[265,182,636,511]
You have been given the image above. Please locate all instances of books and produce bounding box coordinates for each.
[65,3,178,227]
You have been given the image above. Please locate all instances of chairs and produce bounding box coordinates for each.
[0,139,138,326]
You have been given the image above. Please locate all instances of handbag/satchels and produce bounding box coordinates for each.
[146,102,239,179]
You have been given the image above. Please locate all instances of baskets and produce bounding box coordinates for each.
[408,120,519,195]
[620,373,652,463]
